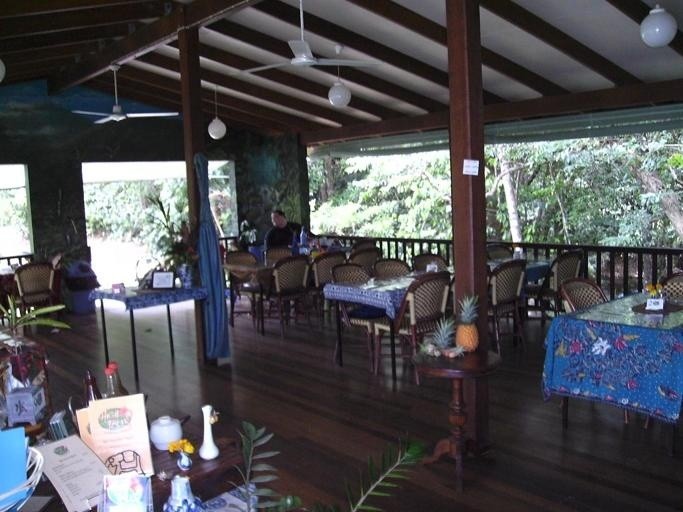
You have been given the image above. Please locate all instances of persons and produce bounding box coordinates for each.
[265,209,307,326]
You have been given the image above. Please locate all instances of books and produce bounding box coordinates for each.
[0,427,27,509]
[48,410,76,443]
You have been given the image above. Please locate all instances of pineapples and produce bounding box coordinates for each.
[455,295,480,353]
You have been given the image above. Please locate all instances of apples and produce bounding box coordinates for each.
[646,283,662,297]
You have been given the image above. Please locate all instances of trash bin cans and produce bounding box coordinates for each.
[66,260,100,315]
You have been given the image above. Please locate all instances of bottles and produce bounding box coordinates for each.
[301,225,308,246]
[82,360,127,406]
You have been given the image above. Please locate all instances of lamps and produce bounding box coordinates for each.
[206,76,227,141]
[326,46,352,108]
[639,0,677,49]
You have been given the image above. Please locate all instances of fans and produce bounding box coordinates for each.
[74,64,179,123]
[240,0,383,73]
